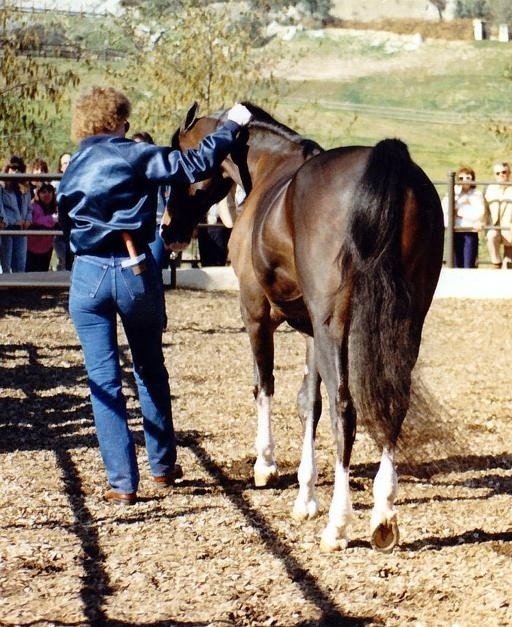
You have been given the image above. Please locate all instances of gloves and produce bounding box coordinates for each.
[227,101,252,126]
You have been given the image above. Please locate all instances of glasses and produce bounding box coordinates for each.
[495,169,509,177]
[457,175,472,183]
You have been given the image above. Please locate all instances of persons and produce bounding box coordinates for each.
[51,78,254,507]
[439,164,485,268]
[483,161,512,269]
[0,132,183,271]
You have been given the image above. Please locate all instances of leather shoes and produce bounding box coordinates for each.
[102,489,139,507]
[154,461,186,487]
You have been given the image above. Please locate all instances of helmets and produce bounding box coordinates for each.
[8,164,26,173]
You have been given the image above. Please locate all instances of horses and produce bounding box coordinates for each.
[160,99,472,553]
